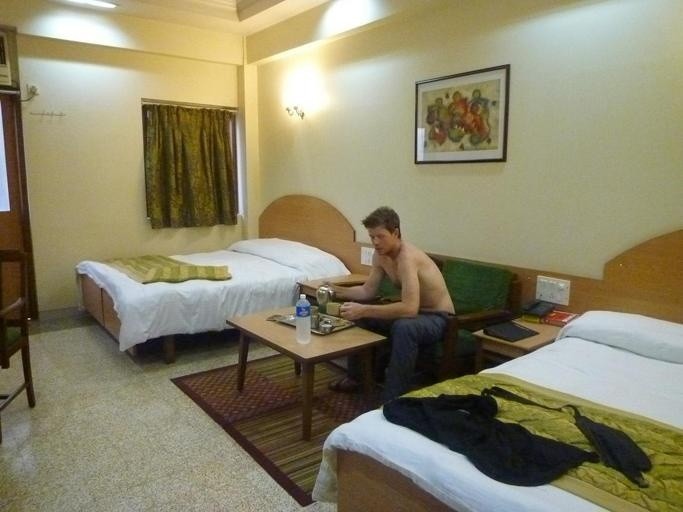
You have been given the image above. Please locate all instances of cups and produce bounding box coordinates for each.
[327,303,344,316]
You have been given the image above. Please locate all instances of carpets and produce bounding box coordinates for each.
[171,353,396,507]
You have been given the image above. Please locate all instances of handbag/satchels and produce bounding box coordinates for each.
[575,407,652,488]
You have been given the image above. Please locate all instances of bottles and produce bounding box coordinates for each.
[310,305,335,334]
[294,293,312,345]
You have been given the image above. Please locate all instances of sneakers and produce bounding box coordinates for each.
[329,375,364,393]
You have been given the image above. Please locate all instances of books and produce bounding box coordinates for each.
[541,309,580,327]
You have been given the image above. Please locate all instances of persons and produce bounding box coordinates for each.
[318,206,457,404]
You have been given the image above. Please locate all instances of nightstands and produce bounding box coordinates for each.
[296,274,369,314]
[471,311,581,374]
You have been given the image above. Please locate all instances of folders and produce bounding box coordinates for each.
[483,320,539,342]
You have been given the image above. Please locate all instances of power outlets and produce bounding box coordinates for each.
[27,83,40,95]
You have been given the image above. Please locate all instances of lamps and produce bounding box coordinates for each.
[283,102,304,121]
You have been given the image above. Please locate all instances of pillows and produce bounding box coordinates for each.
[555,309,683,364]
[227,238,352,282]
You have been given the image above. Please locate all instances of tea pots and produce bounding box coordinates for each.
[315,283,337,315]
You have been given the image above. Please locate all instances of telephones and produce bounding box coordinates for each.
[522,300,555,317]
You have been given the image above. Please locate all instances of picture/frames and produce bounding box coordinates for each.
[414,64,510,164]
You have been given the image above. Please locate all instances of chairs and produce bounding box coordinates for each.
[370,259,523,385]
[353,253,444,305]
[0,249,37,444]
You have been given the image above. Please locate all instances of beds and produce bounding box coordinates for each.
[338,229,683,511]
[76,194,355,365]
